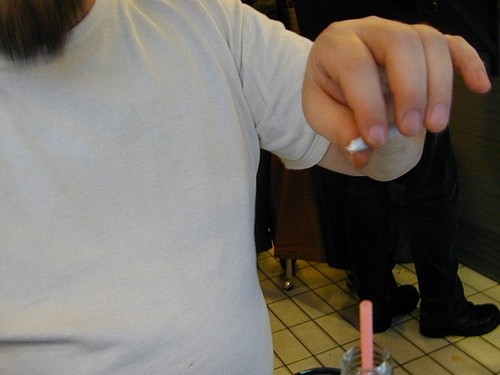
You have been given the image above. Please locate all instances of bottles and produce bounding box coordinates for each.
[342,344,393,375]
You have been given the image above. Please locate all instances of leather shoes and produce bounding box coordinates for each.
[360,285,420,333]
[420,303,500,338]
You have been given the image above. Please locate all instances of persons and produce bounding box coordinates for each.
[292,0,500,338]
[0,0,491,375]
